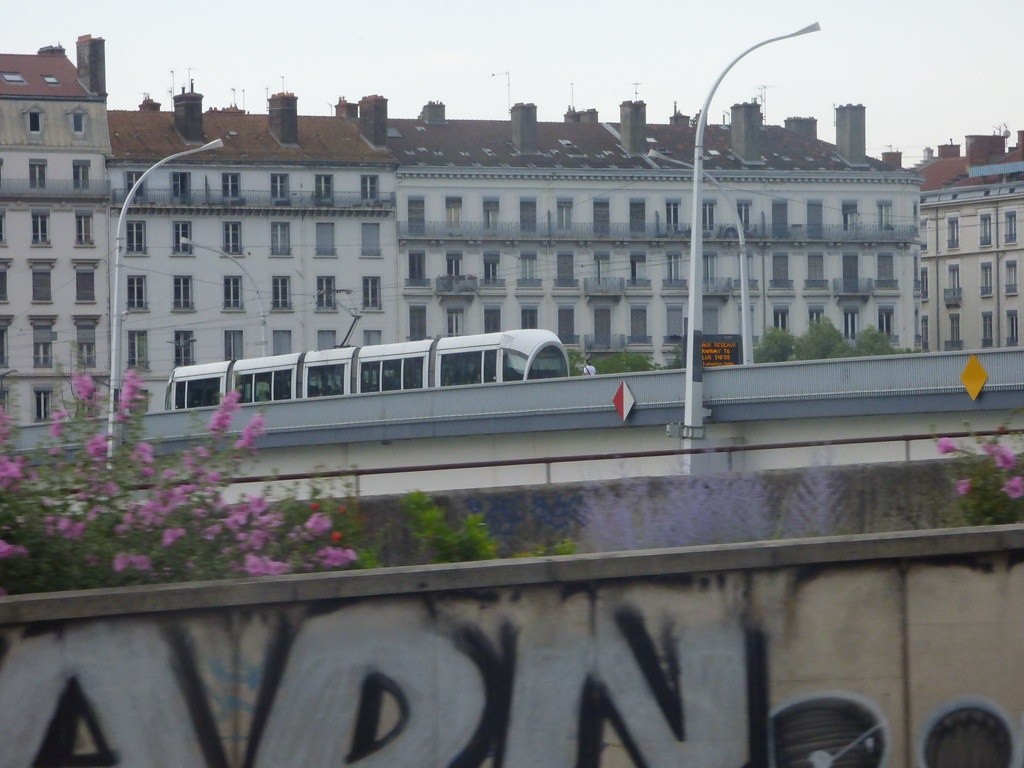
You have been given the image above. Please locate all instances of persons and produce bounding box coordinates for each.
[188,366,561,410]
[583,359,596,376]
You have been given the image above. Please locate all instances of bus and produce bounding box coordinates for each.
[163,328,569,410]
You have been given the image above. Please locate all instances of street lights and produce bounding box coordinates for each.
[682,21,823,477]
[644,150,754,365]
[106,137,226,472]
[179,237,268,358]
